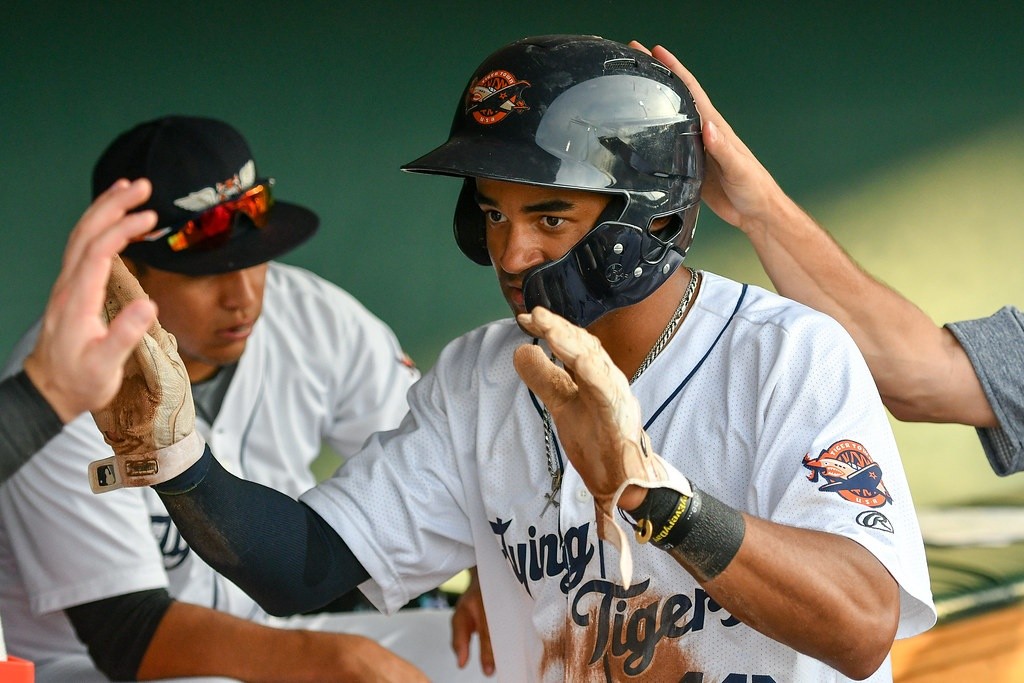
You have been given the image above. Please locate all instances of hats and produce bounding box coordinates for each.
[90,113,320,277]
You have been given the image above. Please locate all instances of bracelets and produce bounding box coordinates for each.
[626,477,745,583]
[647,478,702,551]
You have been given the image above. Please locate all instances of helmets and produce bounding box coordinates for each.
[396,32,709,329]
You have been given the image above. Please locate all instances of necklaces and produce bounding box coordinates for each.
[539,268,697,519]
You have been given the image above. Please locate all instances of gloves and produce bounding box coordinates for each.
[83,253,207,494]
[516,306,693,590]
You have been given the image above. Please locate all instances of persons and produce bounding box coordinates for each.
[630,40,1024,477]
[91,38,938,683]
[0,177,157,490]
[0,115,498,683]
